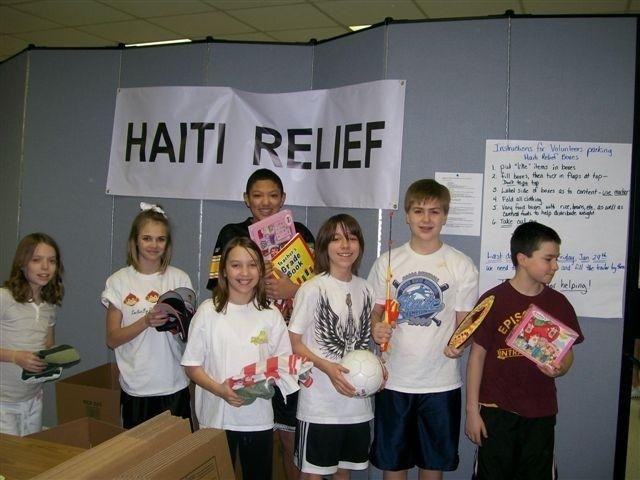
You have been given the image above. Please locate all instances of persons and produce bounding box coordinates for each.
[364,177,481,479]
[181,234,294,479]
[100,202,196,430]
[204,167,316,480]
[0,232,67,437]
[464,220,585,480]
[286,212,388,480]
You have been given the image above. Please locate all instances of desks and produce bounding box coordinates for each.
[1,432,88,480]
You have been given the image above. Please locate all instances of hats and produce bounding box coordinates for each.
[173,286,196,313]
[155,291,189,319]
[150,303,186,340]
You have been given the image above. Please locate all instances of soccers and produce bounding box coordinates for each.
[339,350,384,397]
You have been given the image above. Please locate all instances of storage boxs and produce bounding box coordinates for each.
[21,416,127,450]
[57,360,123,425]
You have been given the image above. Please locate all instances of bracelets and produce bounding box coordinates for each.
[11,351,20,365]
